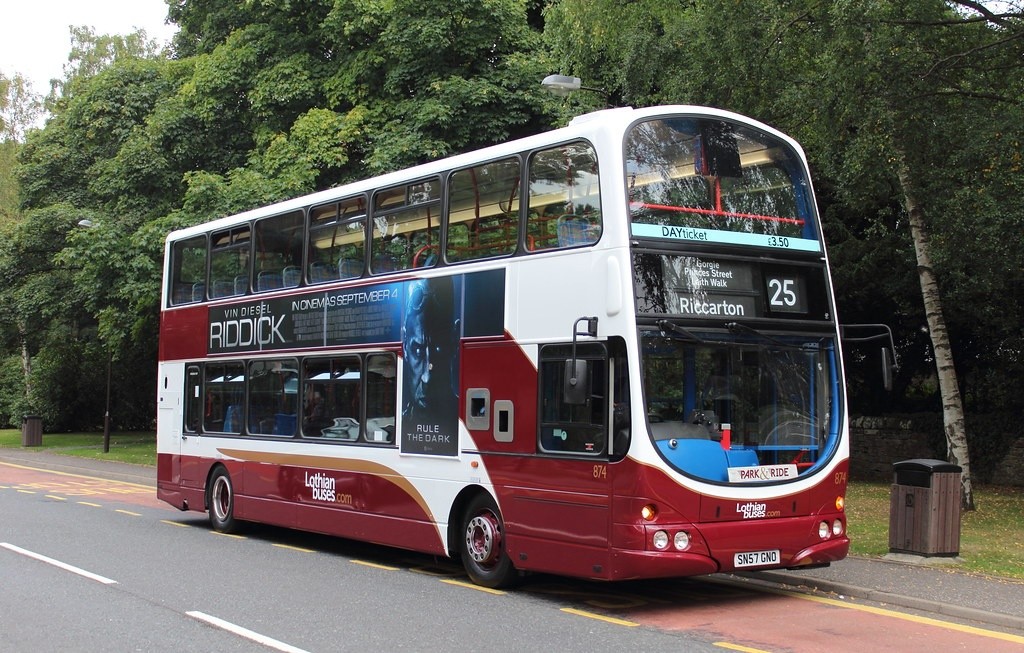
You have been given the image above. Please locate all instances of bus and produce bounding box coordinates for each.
[156,105,898,589]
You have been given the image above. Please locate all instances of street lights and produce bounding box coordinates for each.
[78,220,113,454]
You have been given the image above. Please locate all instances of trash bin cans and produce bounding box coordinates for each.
[21,414,43,447]
[889,458,963,557]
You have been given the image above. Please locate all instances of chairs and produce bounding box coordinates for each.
[177,214,590,303]
[206,403,395,443]
